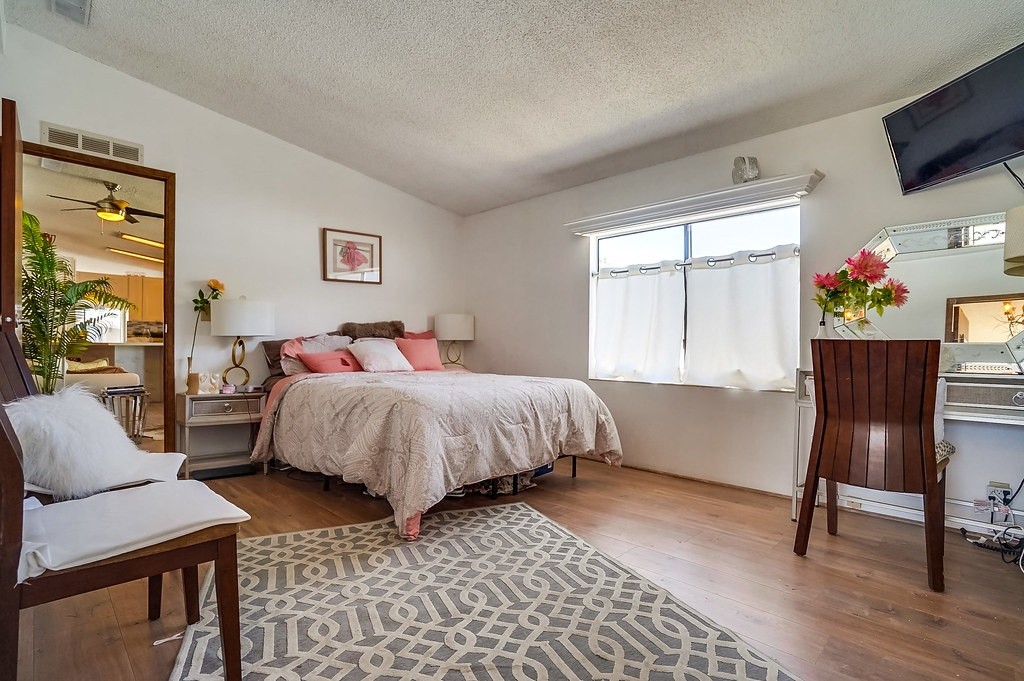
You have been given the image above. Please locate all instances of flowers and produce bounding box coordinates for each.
[187,279,225,373]
[809,247,910,331]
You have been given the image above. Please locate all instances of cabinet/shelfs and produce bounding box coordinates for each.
[174,392,268,481]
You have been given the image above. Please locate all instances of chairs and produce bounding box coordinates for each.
[0,331,187,623]
[793,339,956,593]
[1,402,252,681]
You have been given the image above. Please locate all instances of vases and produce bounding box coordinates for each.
[186,373,199,395]
[815,320,829,339]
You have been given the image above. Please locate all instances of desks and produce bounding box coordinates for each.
[791,369,1024,536]
[98,393,151,445]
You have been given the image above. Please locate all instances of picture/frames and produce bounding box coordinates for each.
[323,228,383,284]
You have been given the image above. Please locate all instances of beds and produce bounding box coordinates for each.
[25,345,140,435]
[248,320,623,541]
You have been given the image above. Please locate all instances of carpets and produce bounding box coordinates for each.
[170,500,806,681]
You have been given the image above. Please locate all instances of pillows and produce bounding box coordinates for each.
[2,381,150,503]
[260,321,445,377]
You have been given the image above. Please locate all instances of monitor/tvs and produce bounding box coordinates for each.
[882,42,1024,196]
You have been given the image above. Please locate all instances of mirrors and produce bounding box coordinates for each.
[944,293,1024,343]
[75,300,129,344]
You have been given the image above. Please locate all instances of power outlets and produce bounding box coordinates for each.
[987,486,1012,505]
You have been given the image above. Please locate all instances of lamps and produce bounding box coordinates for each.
[1001,301,1015,321]
[210,298,275,394]
[434,313,474,370]
[1004,205,1024,277]
[96,209,126,222]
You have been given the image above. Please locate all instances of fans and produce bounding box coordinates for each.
[46,180,164,224]
